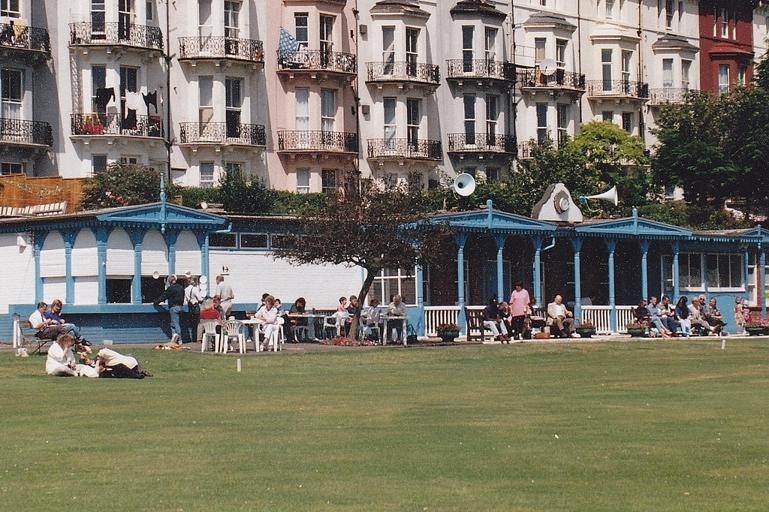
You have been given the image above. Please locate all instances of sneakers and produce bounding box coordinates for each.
[177,338,182,345]
[560,332,568,337]
[497,333,524,342]
[570,331,582,339]
[140,368,153,377]
[171,333,178,343]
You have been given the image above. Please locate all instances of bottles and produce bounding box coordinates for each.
[236,359,243,371]
[721,339,726,350]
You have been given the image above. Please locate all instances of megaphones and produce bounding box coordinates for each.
[580,184,619,208]
[450,172,476,197]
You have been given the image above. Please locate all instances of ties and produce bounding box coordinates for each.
[40,314,45,323]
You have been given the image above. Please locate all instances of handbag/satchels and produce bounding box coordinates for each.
[521,317,532,338]
[407,334,418,344]
[188,299,200,315]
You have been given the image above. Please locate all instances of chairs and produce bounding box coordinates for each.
[200,317,285,354]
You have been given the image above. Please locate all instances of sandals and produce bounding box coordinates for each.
[258,338,273,353]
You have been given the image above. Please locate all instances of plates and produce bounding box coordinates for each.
[153,271,161,280]
[199,275,209,298]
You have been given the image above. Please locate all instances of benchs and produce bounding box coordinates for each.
[18,320,56,356]
[464,307,575,342]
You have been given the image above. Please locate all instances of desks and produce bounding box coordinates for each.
[361,316,408,346]
[287,313,327,343]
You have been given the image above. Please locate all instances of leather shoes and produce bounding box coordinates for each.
[83,339,92,347]
[659,322,729,339]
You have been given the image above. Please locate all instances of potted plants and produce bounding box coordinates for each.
[745,322,764,336]
[407,323,418,344]
[627,320,646,337]
[437,324,459,342]
[576,322,596,338]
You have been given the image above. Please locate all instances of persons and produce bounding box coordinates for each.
[42,298,80,335]
[27,302,93,353]
[156,273,234,343]
[253,294,308,352]
[43,335,151,377]
[635,294,728,338]
[735,296,748,333]
[583,288,608,305]
[484,281,580,340]
[337,295,407,344]
